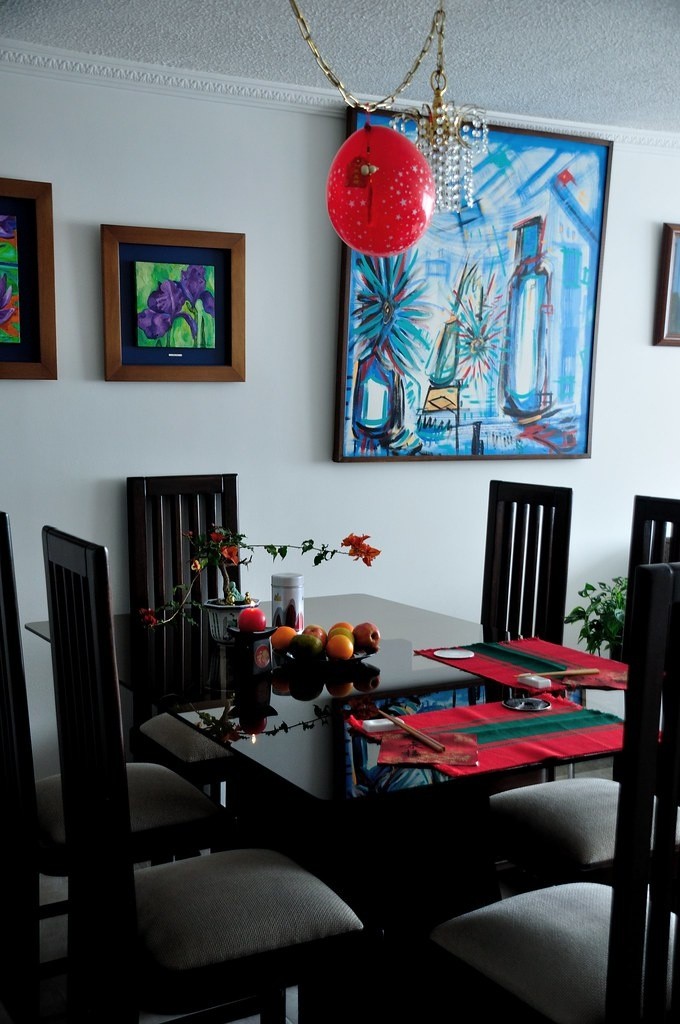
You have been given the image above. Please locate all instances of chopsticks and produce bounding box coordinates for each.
[378,709,446,753]
[512,668,600,677]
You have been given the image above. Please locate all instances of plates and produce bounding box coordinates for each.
[433,649,474,659]
[502,698,551,712]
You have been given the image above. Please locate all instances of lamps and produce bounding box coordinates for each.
[391,67,490,214]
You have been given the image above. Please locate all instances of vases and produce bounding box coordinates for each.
[203,598,261,646]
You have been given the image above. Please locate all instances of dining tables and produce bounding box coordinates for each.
[23,593,662,1023]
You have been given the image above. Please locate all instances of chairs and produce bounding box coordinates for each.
[1,475,680,1024]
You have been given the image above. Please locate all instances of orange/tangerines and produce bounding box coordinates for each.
[326,635,353,659]
[329,623,354,633]
[328,628,354,646]
[290,635,322,657]
[326,682,353,696]
[289,685,323,701]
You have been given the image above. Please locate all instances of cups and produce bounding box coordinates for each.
[271,573,305,634]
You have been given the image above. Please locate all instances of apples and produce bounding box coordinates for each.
[271,627,296,649]
[353,623,380,648]
[238,609,266,631]
[354,676,380,692]
[239,717,267,734]
[302,625,327,646]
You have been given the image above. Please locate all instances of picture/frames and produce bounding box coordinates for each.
[329,679,503,802]
[651,223,680,349]
[101,223,247,383]
[0,177,59,381]
[333,104,613,464]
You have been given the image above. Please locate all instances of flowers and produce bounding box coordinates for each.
[134,520,383,630]
